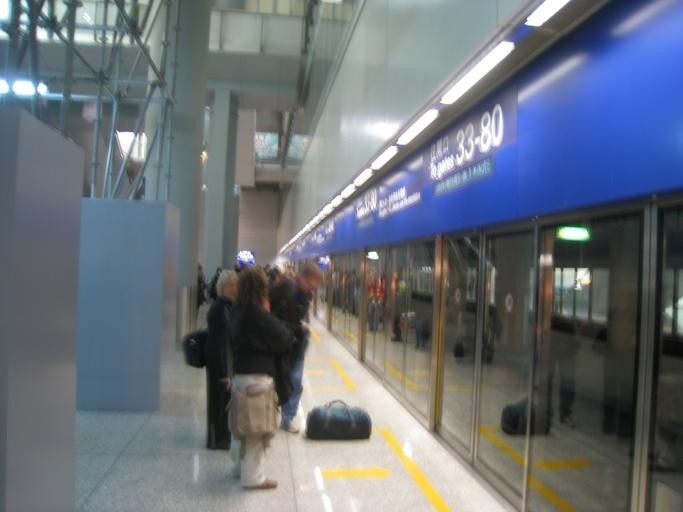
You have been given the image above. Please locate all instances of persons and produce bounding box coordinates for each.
[325,266,407,342]
[538,330,582,433]
[198,263,321,489]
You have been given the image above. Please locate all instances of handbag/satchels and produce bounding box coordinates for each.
[231,374,279,437]
[501,398,553,435]
[305,399,372,439]
[183,321,212,368]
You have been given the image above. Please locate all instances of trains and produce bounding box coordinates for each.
[286,0,681,511]
